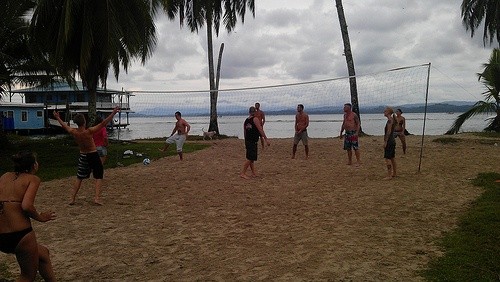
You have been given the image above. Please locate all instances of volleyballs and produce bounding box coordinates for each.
[143,158,151,165]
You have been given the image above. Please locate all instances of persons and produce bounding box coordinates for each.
[240,107,271,179]
[0,152,57,282]
[53,106,120,206]
[93,117,108,165]
[383,107,406,180]
[291,105,309,160]
[340,103,363,165]
[159,112,190,160]
[255,103,266,152]
